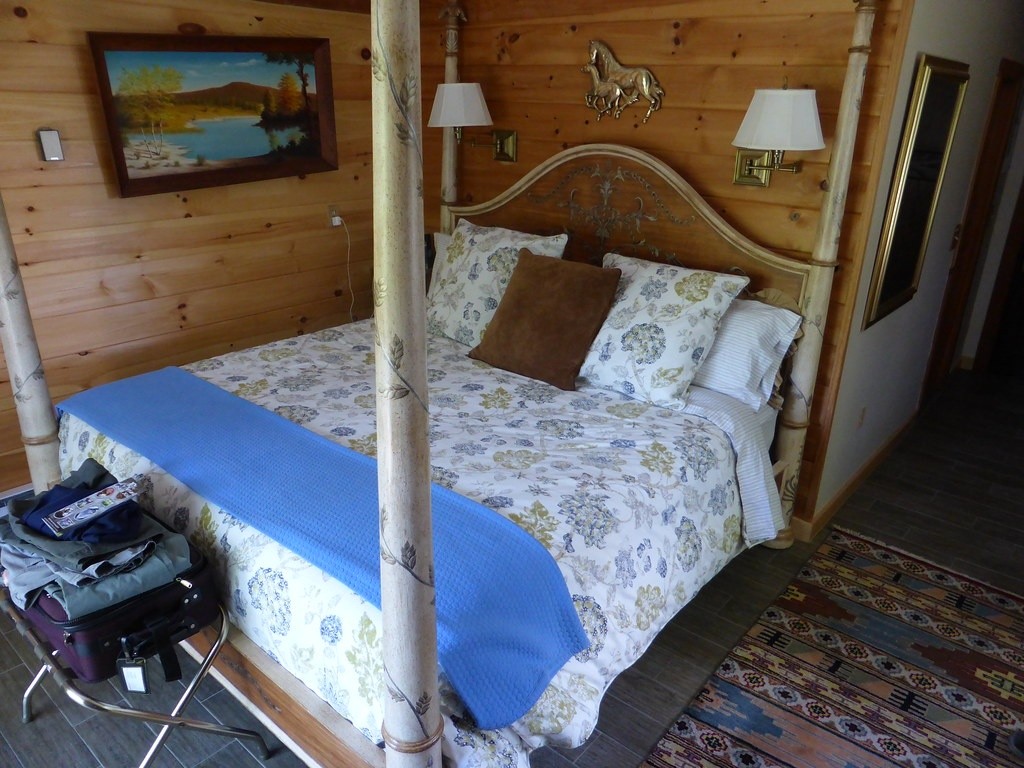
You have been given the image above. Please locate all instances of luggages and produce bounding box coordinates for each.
[6,502,223,683]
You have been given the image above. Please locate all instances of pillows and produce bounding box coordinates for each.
[576,250,751,413]
[464,247,622,393]
[689,300,805,408]
[426,219,571,347]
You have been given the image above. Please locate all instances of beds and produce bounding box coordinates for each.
[46,141,838,768]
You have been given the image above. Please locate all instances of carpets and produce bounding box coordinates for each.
[635,522,1024,768]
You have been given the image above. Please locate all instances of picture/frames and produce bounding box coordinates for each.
[859,54,972,331]
[86,31,339,201]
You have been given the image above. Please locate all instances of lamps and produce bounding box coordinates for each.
[427,82,519,163]
[727,75,827,188]
